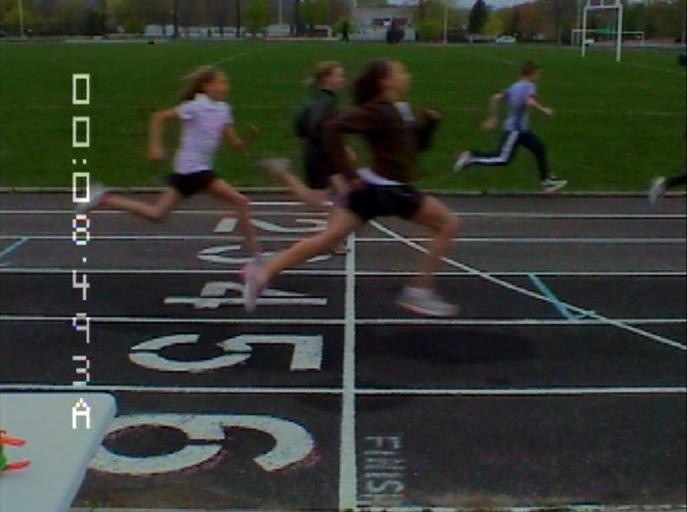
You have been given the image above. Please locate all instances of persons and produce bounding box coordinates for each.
[76,64,262,256]
[253,61,358,256]
[649,174,687,207]
[452,59,568,195]
[237,57,464,319]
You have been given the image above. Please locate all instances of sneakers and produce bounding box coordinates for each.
[397,292,458,318]
[76,188,108,215]
[453,151,470,172]
[650,177,666,204]
[542,178,567,194]
[241,263,265,310]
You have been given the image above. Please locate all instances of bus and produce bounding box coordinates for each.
[371,18,390,27]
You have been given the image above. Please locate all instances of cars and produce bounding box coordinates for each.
[464,31,516,44]
